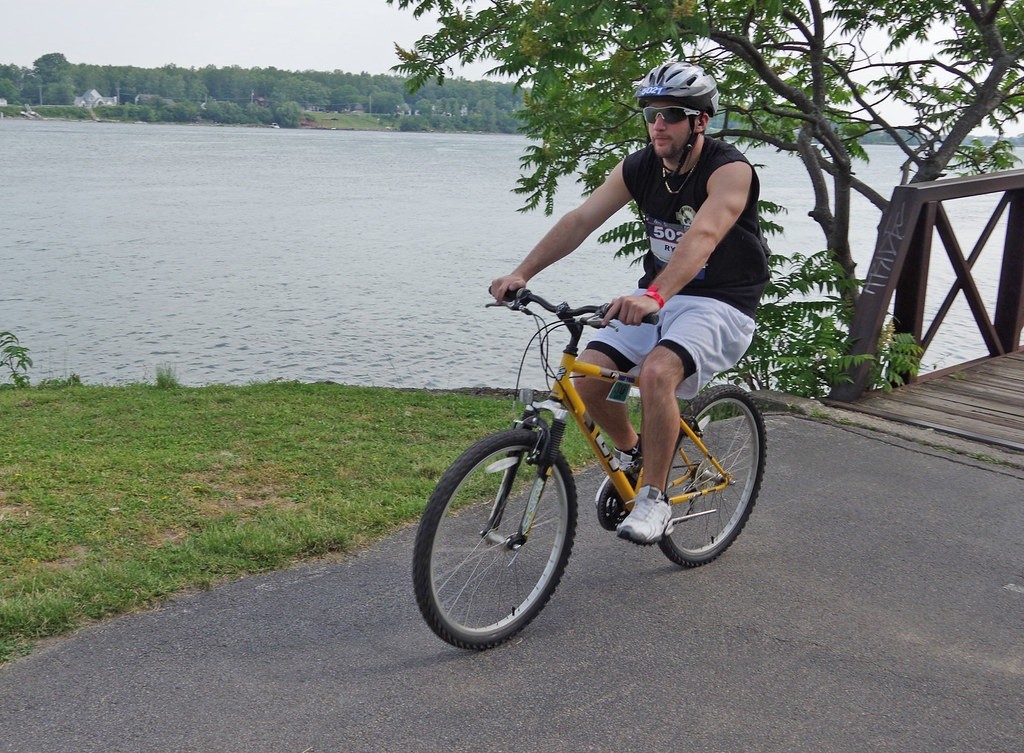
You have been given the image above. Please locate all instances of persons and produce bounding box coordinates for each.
[490,64,772,544]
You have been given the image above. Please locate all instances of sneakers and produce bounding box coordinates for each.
[594,447,637,510]
[616,485,675,545]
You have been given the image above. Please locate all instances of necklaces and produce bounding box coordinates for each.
[661,156,699,194]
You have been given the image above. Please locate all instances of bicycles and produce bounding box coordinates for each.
[412,286,769,653]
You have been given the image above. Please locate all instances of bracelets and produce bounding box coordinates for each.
[642,285,665,309]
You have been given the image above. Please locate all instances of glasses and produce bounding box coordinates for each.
[642,106,704,123]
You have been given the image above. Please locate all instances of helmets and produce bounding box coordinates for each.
[633,62,719,118]
